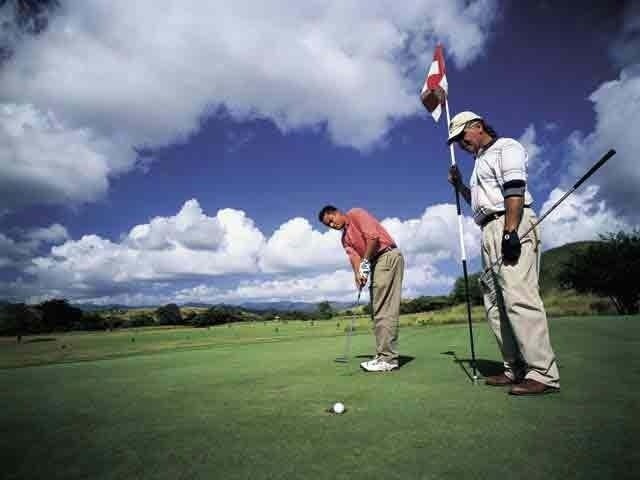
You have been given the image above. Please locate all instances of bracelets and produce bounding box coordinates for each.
[362,258,370,264]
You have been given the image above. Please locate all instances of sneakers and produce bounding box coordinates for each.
[375,355,399,368]
[360,358,392,372]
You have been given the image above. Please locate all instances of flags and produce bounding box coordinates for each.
[419,43,449,125]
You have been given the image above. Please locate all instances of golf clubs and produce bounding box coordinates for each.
[334,279,363,363]
[478,149,616,294]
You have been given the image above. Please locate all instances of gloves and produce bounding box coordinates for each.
[502,230,521,262]
[449,166,466,193]
[359,260,370,278]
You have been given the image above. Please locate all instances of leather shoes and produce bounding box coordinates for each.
[486,374,522,386]
[511,379,560,395]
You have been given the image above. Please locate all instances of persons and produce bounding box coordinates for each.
[318,205,404,372]
[446,110,561,397]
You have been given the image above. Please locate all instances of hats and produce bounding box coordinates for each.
[447,111,481,145]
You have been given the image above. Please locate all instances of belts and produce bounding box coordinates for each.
[370,244,397,264]
[480,205,531,225]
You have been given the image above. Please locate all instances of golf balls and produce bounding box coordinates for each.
[333,402,343,413]
